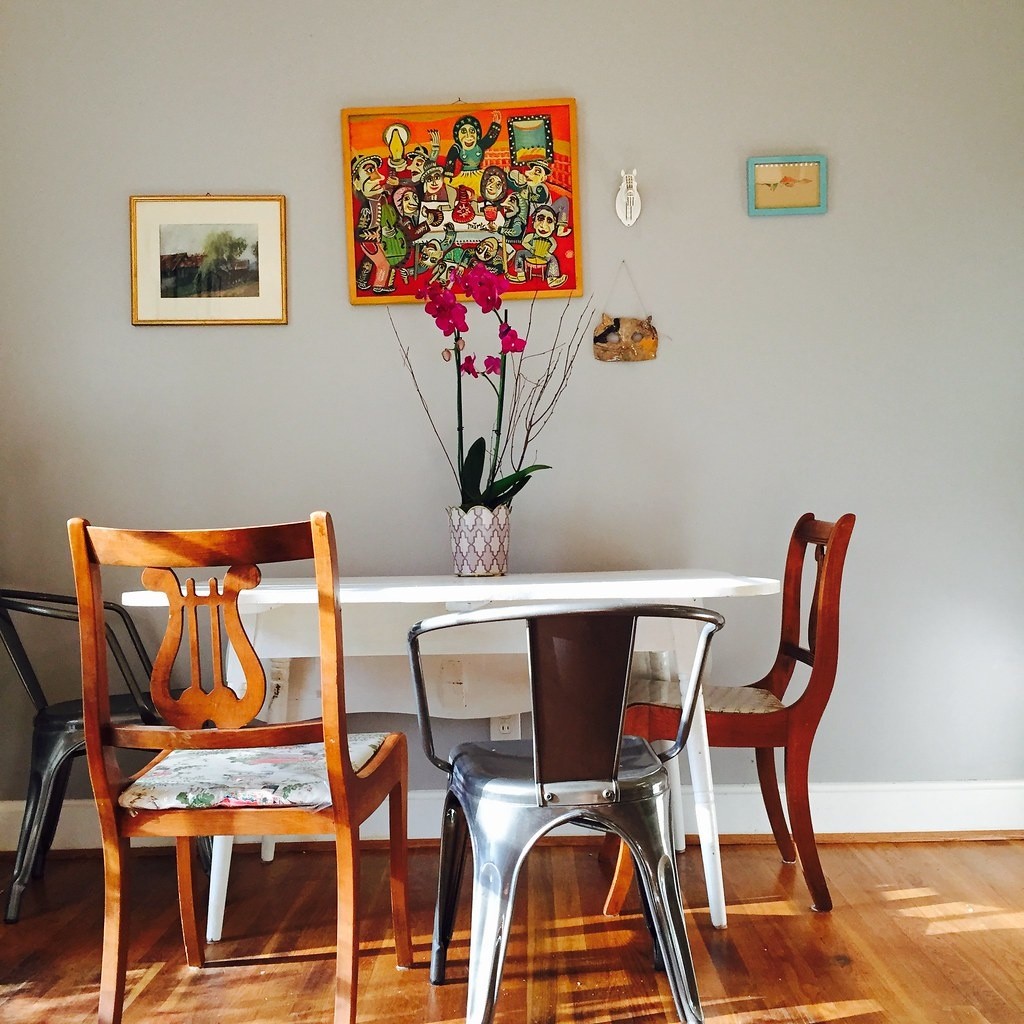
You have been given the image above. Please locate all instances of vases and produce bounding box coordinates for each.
[442,505,512,576]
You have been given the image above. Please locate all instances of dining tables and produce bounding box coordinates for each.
[119,566,783,947]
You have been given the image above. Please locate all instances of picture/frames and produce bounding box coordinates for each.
[747,154,829,217]
[340,97,583,307]
[129,195,288,327]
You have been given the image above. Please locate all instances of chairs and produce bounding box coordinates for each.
[404,604,725,1024]
[66,510,413,1024]
[0,588,239,925]
[596,510,857,919]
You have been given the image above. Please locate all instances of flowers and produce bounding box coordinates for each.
[379,262,595,517]
